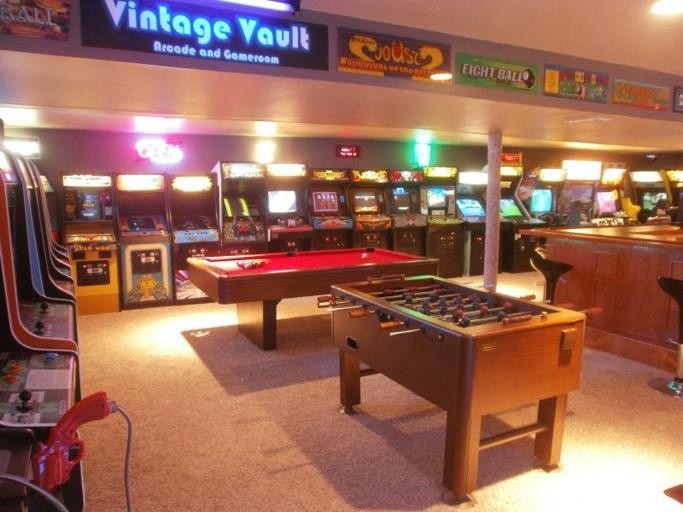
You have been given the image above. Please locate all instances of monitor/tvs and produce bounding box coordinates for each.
[456,197,486,217]
[265,189,303,220]
[352,191,380,214]
[390,189,418,212]
[171,196,213,228]
[529,187,553,215]
[310,189,347,216]
[222,194,251,219]
[126,216,157,231]
[76,191,102,219]
[639,189,668,213]
[500,197,524,218]
[596,190,617,217]
[426,187,448,209]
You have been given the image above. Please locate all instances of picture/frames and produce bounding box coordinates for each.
[672,87,683,113]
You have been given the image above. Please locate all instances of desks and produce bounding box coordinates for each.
[185,248,440,353]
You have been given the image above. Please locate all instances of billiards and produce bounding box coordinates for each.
[361,253,368,259]
[237,260,265,269]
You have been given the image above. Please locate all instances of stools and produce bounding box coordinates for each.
[657,275,683,395]
[529,248,574,305]
